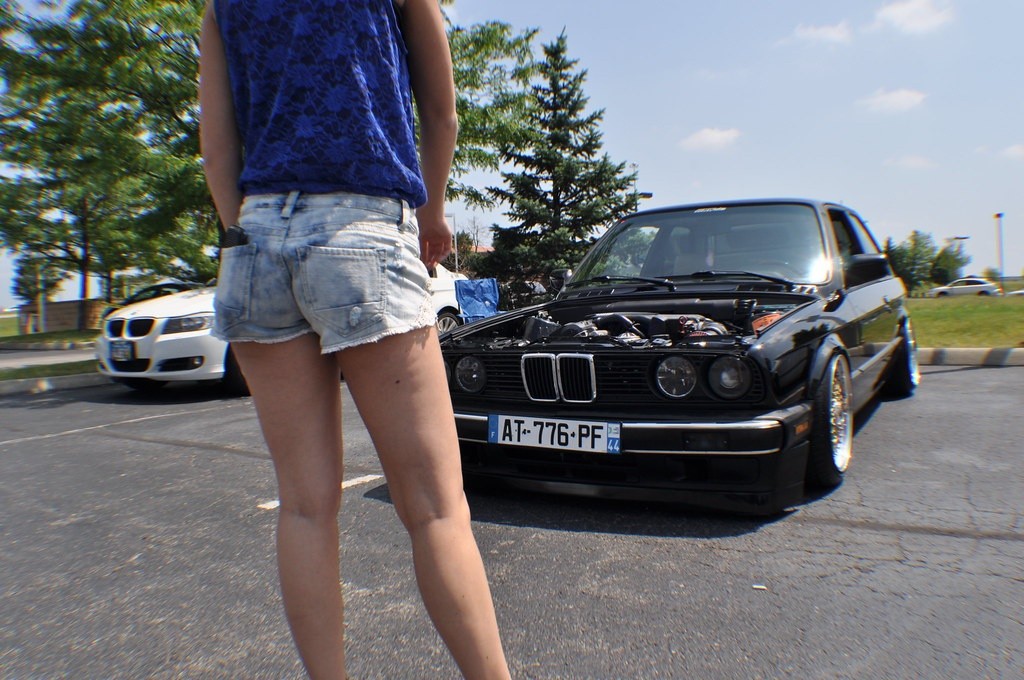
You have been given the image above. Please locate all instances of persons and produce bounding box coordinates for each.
[200,0,512,680]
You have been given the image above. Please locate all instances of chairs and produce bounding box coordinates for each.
[664,235,706,273]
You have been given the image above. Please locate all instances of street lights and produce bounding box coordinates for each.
[995,212,1006,278]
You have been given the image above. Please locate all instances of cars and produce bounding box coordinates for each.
[428,198,921,493]
[95,250,474,397]
[929,274,1004,298]
